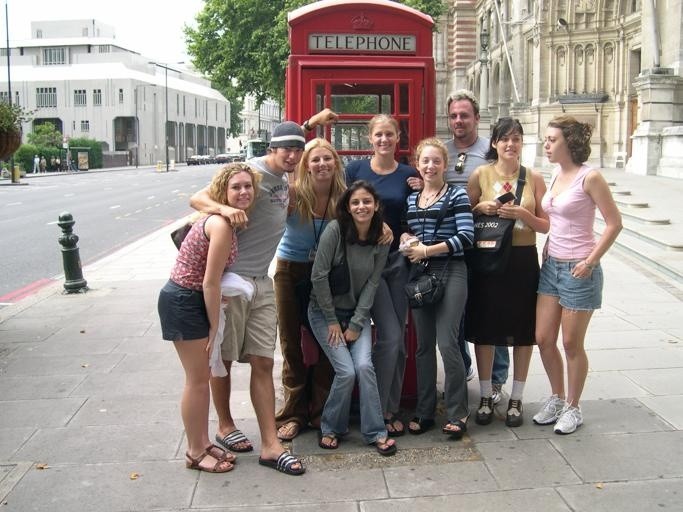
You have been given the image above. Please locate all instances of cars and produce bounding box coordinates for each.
[187,153,246,165]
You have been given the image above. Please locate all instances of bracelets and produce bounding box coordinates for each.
[583,259,595,271]
[422,246,431,260]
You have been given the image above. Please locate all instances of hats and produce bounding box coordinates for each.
[269,121,306,149]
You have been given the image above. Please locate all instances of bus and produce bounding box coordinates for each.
[246,138,270,160]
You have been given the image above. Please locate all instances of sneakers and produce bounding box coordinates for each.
[506,399,523,427]
[475,396,494,424]
[553,402,583,434]
[466,367,474,381]
[491,383,503,404]
[533,394,567,425]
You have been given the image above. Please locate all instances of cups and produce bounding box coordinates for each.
[409,241,421,264]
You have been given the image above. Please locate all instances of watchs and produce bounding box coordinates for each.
[302,119,316,131]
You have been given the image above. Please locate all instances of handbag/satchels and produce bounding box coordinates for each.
[170,223,192,249]
[328,262,351,296]
[404,272,444,308]
[471,215,516,273]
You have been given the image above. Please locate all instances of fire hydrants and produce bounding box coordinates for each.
[57,211,87,293]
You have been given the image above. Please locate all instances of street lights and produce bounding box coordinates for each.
[136,83,157,168]
[147,61,183,172]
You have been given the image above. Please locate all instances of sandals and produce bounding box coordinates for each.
[185,450,234,473]
[408,417,436,435]
[442,410,471,438]
[205,443,236,462]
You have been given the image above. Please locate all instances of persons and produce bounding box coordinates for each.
[443,89,511,405]
[306,178,398,456]
[33,154,61,173]
[156,159,264,475]
[531,114,624,436]
[465,115,551,428]
[189,106,340,474]
[399,136,474,436]
[274,136,349,441]
[345,113,426,435]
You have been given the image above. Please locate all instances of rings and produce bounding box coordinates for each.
[332,333,336,336]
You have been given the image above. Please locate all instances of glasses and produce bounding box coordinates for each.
[455,152,467,173]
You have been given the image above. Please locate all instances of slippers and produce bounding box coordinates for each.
[215,430,253,452]
[319,433,339,449]
[277,421,299,440]
[369,437,397,455]
[259,450,306,475]
[383,411,405,436]
